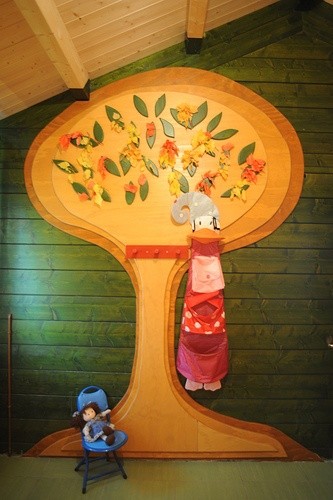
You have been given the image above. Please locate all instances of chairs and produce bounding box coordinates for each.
[74,386,127,494]
